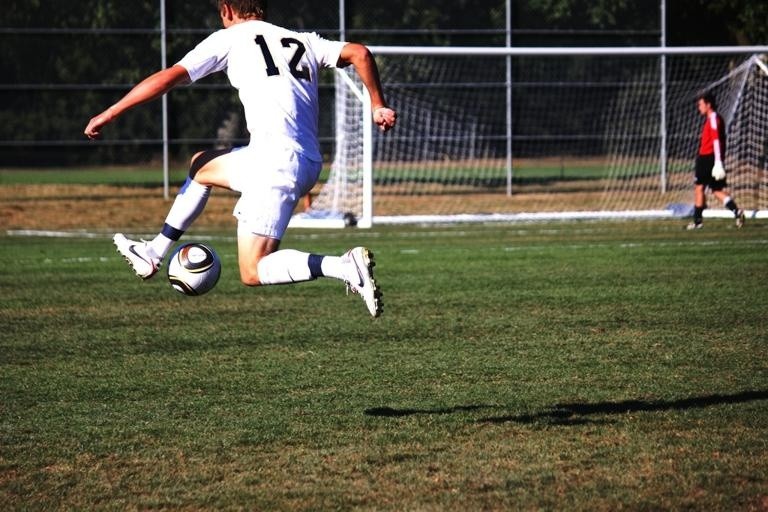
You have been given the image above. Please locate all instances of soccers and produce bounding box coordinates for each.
[167,243,221,295]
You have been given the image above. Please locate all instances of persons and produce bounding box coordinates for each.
[683,93,748,235]
[80,1,400,320]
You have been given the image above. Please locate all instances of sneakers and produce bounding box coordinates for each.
[681,221,703,232]
[112,232,163,283]
[338,245,386,318]
[733,206,745,231]
